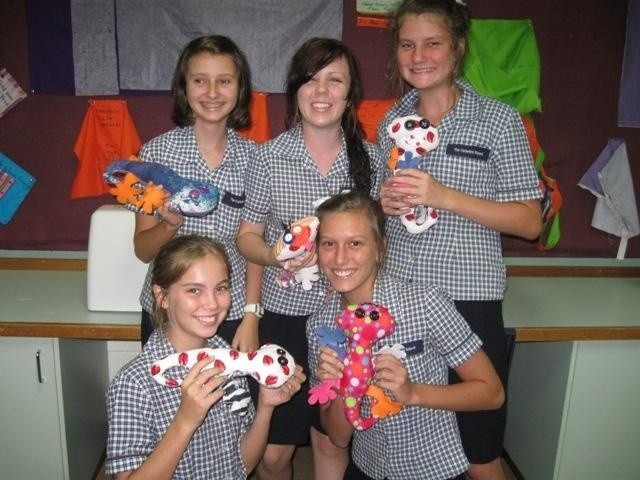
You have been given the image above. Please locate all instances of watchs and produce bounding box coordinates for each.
[242,304,267,318]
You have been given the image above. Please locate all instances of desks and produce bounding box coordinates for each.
[0,257,640,480]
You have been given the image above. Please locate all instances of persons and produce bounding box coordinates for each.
[302,190,509,480]
[236,34,386,480]
[130,29,266,408]
[102,232,307,480]
[373,0,545,480]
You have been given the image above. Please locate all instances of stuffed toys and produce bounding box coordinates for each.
[147,341,297,388]
[100,153,221,220]
[307,302,405,434]
[273,213,321,292]
[383,112,440,235]
